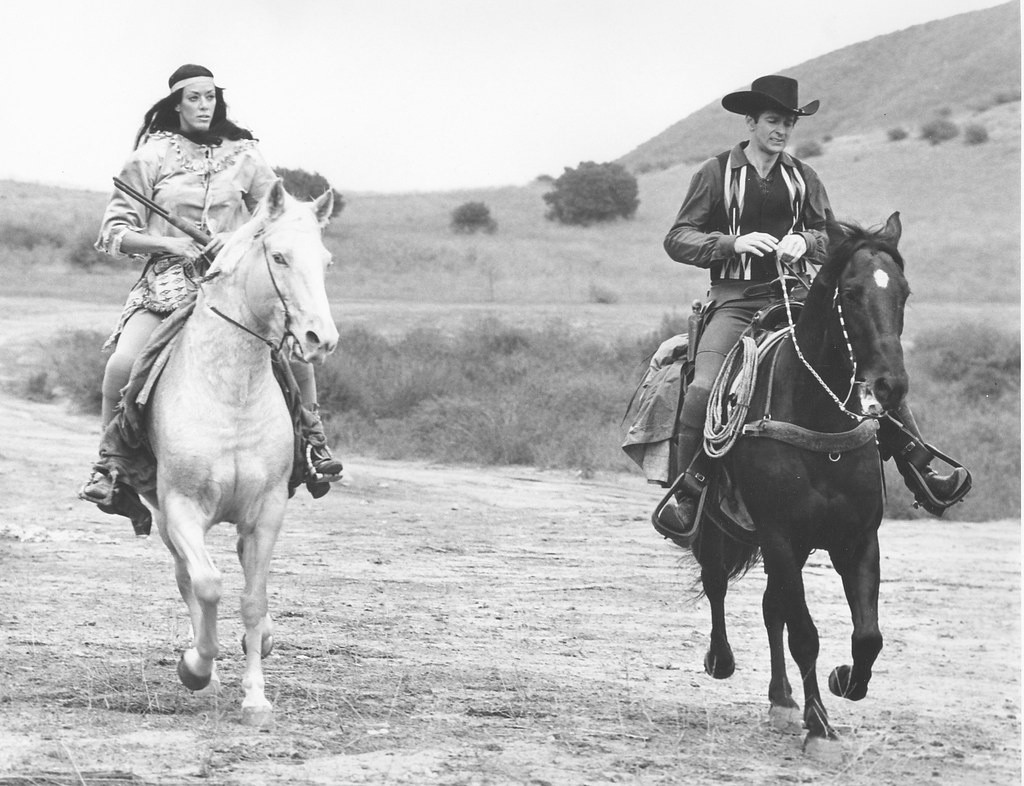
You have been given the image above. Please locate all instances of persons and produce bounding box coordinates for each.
[82,61,344,515]
[657,74,968,547]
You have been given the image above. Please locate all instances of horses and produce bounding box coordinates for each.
[657,208,912,761]
[133,178,340,728]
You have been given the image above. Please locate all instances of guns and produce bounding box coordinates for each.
[112,176,213,246]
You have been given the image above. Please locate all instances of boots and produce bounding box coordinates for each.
[659,434,710,547]
[876,400,968,517]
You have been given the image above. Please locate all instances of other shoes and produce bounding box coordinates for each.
[85,471,117,514]
[300,443,343,499]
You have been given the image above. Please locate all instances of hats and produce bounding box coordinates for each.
[721,76,820,115]
